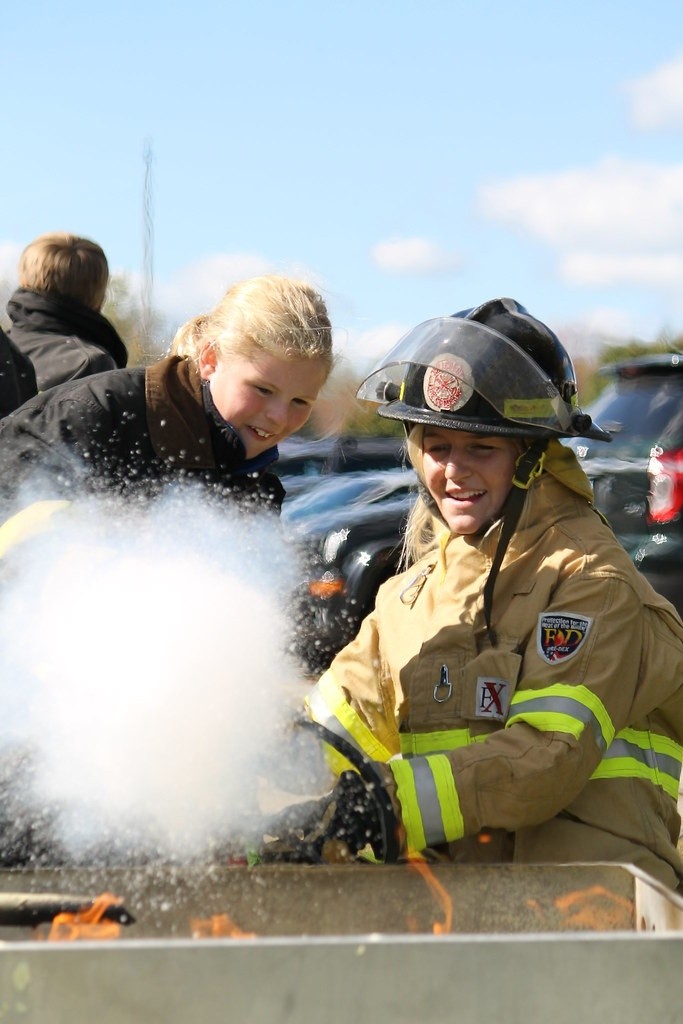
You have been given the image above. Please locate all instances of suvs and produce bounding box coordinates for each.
[549,349,683,612]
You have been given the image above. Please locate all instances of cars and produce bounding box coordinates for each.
[276,438,407,475]
[273,463,471,630]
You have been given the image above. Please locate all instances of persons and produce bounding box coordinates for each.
[312,296,683,891]
[4,233,128,392]
[0,279,333,530]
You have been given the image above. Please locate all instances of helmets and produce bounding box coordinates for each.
[376,299,614,443]
